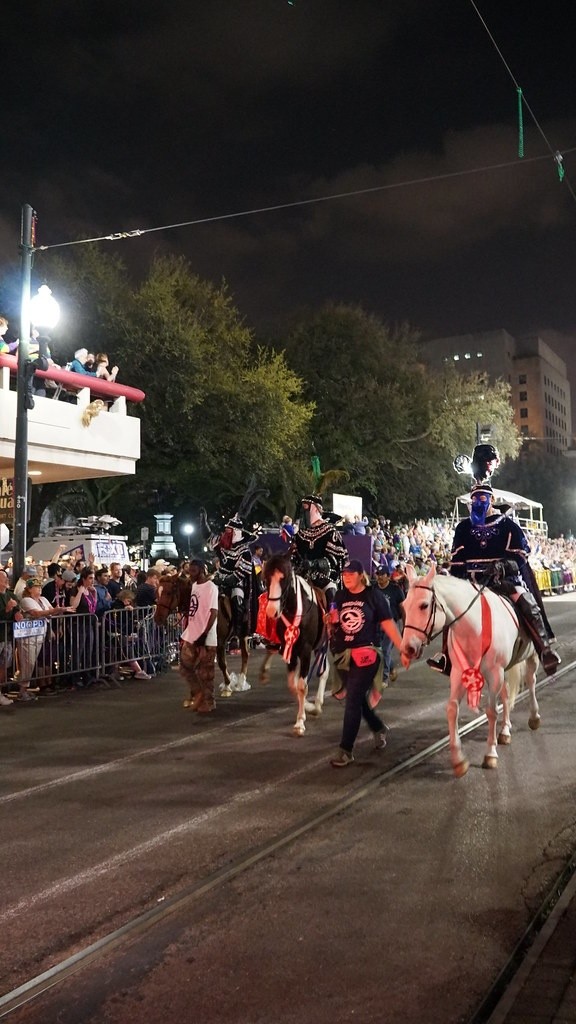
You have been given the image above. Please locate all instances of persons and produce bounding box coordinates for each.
[343,515,356,535]
[0,545,190,706]
[280,515,294,542]
[287,494,349,602]
[354,515,368,535]
[181,560,218,711]
[375,565,405,689]
[0,317,19,355]
[366,515,459,576]
[522,529,576,595]
[199,507,252,648]
[425,485,561,675]
[251,546,263,565]
[16,329,52,397]
[323,560,410,768]
[64,348,119,412]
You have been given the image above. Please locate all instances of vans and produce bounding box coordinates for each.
[25,514,128,572]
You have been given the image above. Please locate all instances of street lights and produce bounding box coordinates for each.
[182,523,194,559]
[9,203,62,593]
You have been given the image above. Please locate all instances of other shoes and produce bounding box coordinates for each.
[0,642,267,706]
[198,697,217,713]
[189,694,204,711]
[389,668,398,681]
[381,681,389,689]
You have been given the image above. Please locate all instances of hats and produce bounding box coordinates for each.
[374,564,389,575]
[301,456,350,509]
[168,565,177,571]
[452,444,500,499]
[62,571,78,582]
[156,559,170,566]
[126,562,140,570]
[24,578,43,589]
[225,474,270,531]
[339,559,363,574]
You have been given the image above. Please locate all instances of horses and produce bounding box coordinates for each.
[400,563,542,780]
[153,571,274,709]
[261,543,342,737]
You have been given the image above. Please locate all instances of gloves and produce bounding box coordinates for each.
[483,560,505,587]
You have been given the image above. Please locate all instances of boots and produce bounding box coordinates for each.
[230,594,249,647]
[426,625,452,676]
[516,592,561,668]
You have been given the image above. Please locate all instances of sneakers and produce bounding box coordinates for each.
[373,723,389,749]
[330,751,355,766]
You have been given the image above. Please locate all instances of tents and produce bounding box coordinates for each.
[451,488,544,529]
